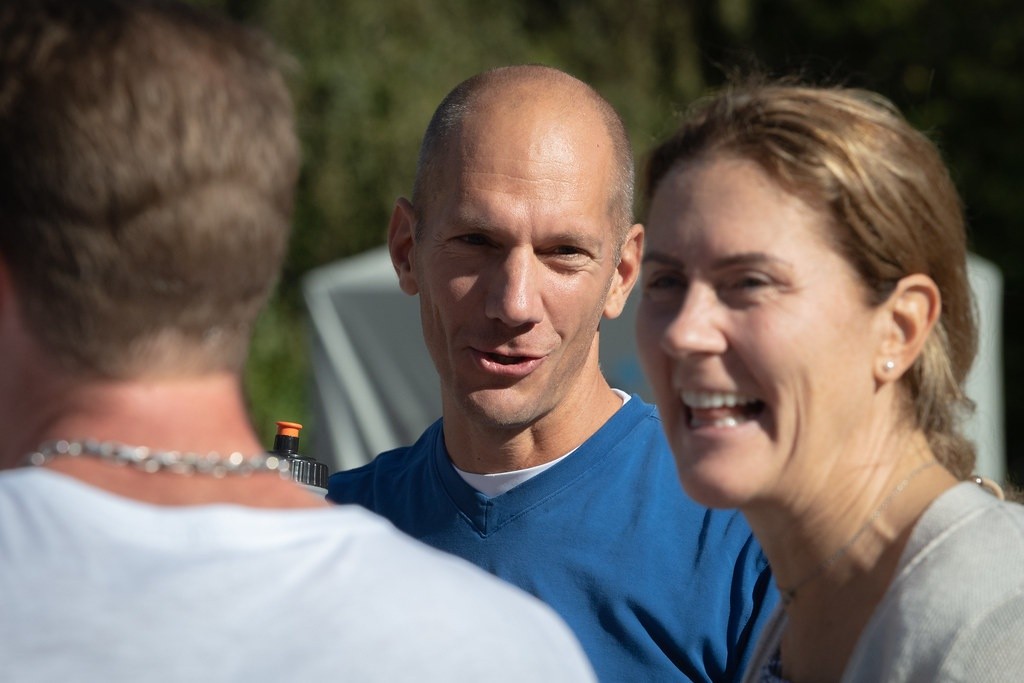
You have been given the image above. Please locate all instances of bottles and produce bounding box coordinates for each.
[266,421,328,499]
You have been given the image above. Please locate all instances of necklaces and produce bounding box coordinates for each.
[13,434,298,480]
[756,455,941,595]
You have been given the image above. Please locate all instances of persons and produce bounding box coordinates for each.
[0,0,605,683]
[633,53,1024,682]
[304,62,780,683]
